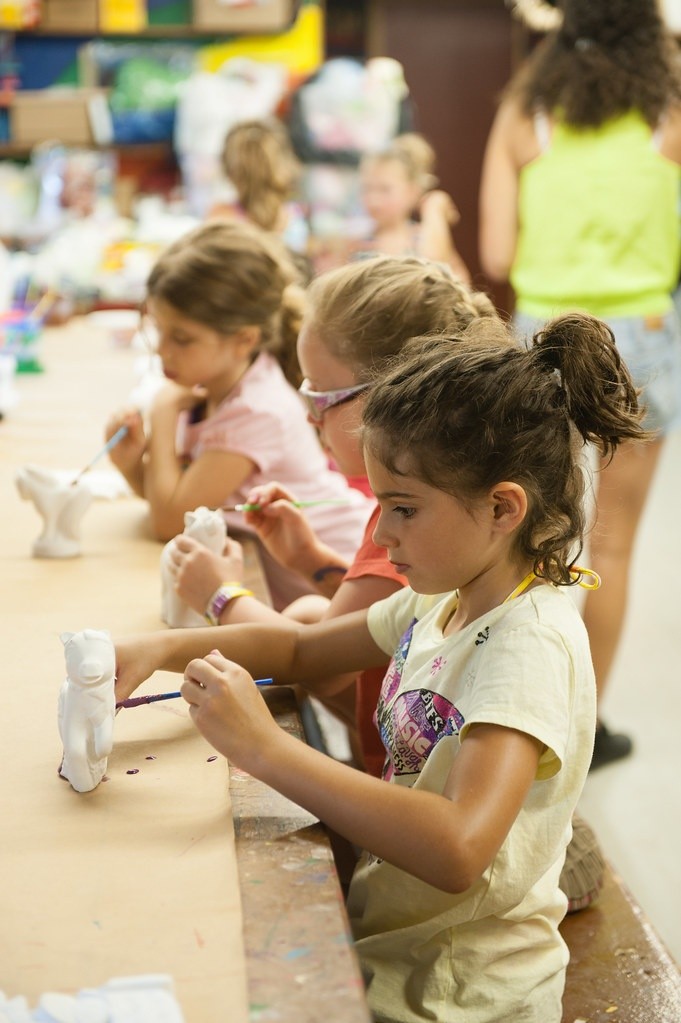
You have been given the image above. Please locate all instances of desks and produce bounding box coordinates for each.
[2,311,374,1021]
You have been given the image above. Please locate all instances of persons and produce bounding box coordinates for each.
[105,222,374,611]
[478,0,681,768]
[210,120,472,292]
[113,313,655,1023]
[170,258,604,911]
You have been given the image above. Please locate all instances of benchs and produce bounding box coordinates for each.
[555,871,680,1022]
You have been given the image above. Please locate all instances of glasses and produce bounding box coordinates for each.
[298,380,371,421]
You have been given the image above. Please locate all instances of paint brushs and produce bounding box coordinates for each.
[115,677,274,710]
[71,424,128,488]
[210,500,342,513]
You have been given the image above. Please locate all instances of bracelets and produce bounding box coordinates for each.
[206,584,254,625]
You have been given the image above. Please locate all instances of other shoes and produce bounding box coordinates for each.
[559,818,606,912]
[590,723,632,771]
